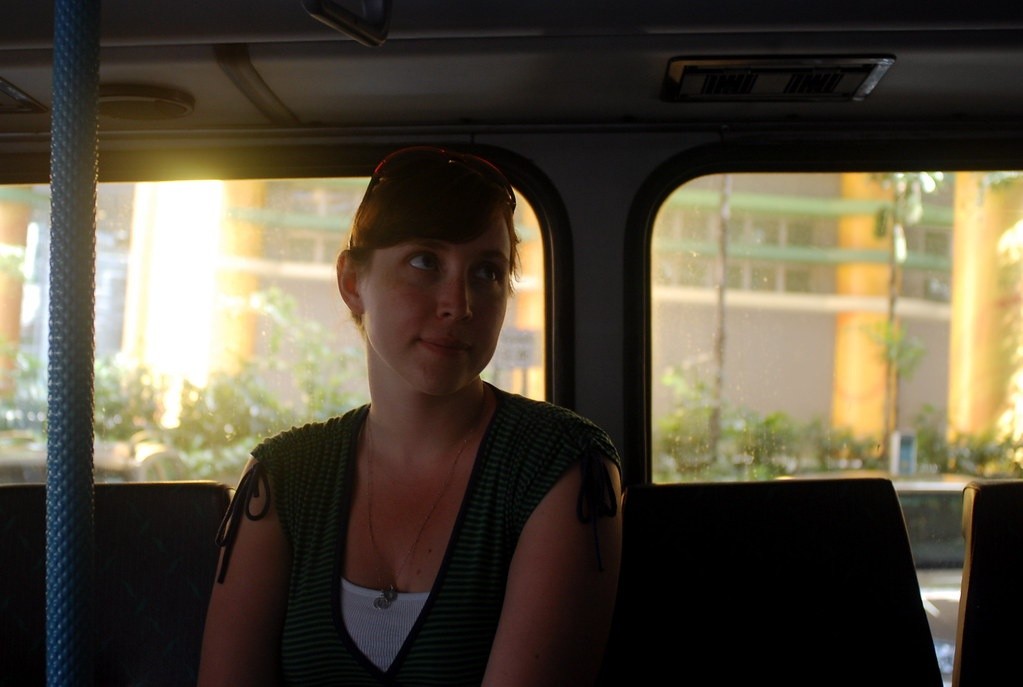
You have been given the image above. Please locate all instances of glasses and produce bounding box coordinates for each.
[366,146,517,214]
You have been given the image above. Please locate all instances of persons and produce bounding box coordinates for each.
[194,146,628,687]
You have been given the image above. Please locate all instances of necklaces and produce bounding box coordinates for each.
[365,396,487,609]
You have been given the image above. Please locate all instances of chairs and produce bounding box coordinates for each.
[0,471,1023,687]
